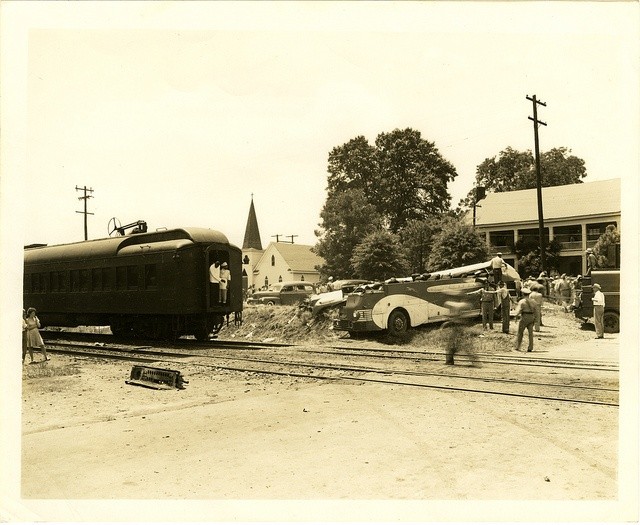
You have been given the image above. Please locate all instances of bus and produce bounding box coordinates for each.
[333,273,523,337]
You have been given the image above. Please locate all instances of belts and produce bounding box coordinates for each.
[524,312,533,314]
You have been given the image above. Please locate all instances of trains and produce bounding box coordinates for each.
[23,221,244,342]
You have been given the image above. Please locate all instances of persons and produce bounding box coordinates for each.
[482,281,510,334]
[490,252,507,284]
[209,261,225,307]
[515,288,534,352]
[25,307,51,364]
[464,280,497,331]
[327,276,333,286]
[22,309,29,364]
[524,271,582,313]
[529,285,544,326]
[585,248,596,275]
[388,276,399,284]
[218,262,231,303]
[592,283,605,339]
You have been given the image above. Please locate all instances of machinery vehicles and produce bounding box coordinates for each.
[573,240,620,333]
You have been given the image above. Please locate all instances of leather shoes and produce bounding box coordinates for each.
[595,336,602,339]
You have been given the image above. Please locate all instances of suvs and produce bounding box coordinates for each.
[247,281,316,307]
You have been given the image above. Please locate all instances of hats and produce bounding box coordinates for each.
[592,283,603,289]
[521,288,531,294]
